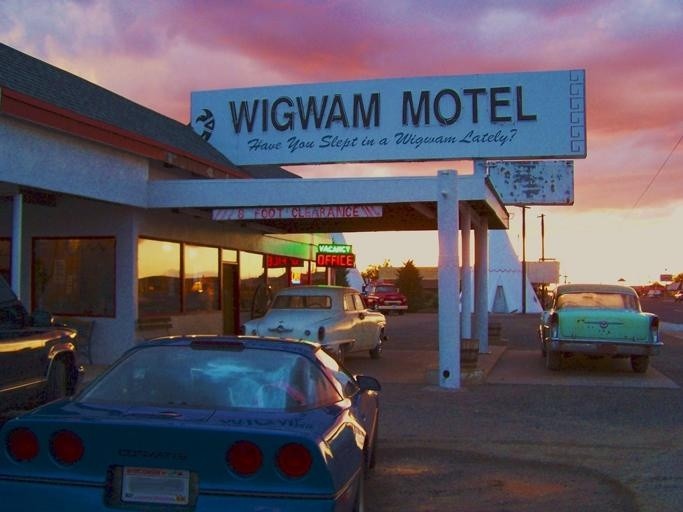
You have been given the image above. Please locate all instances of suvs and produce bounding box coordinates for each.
[1,277,89,414]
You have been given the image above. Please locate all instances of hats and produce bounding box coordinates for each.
[538,284,664,372]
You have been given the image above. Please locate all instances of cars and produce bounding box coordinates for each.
[0,333,383,512]
[239,284,390,365]
[538,284,664,372]
[360,278,408,316]
